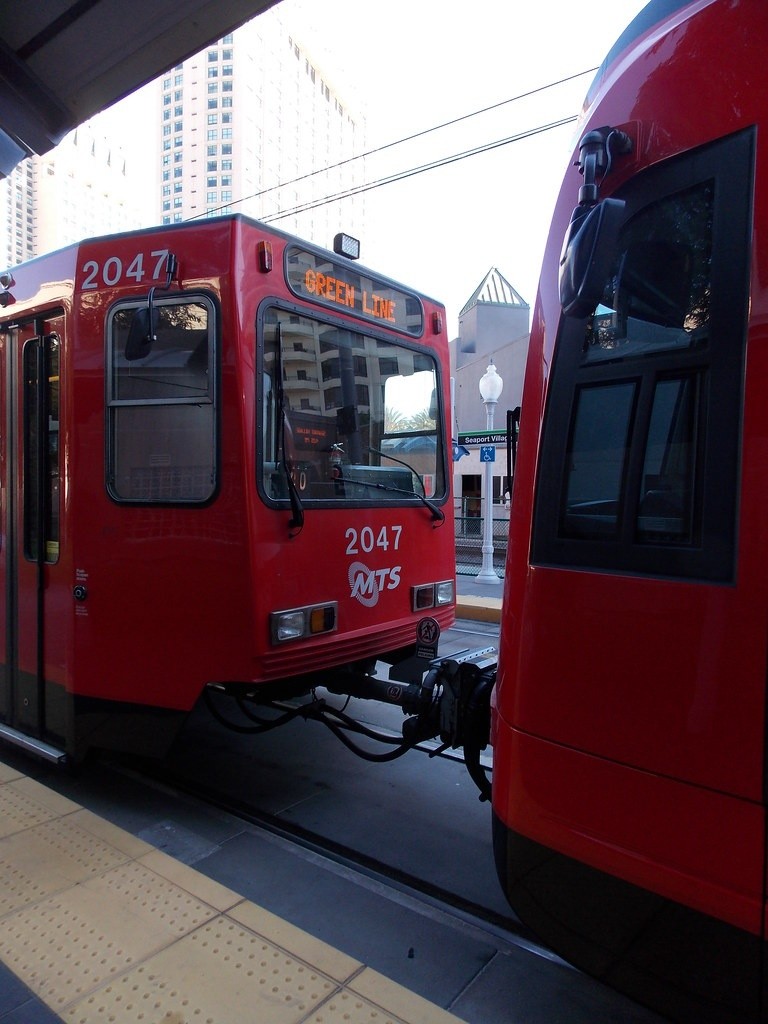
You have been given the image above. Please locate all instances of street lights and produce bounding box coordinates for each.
[474,357,504,586]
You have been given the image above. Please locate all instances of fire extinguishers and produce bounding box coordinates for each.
[330,442,345,478]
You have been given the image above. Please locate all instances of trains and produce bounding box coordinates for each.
[0,0,768,1024]
